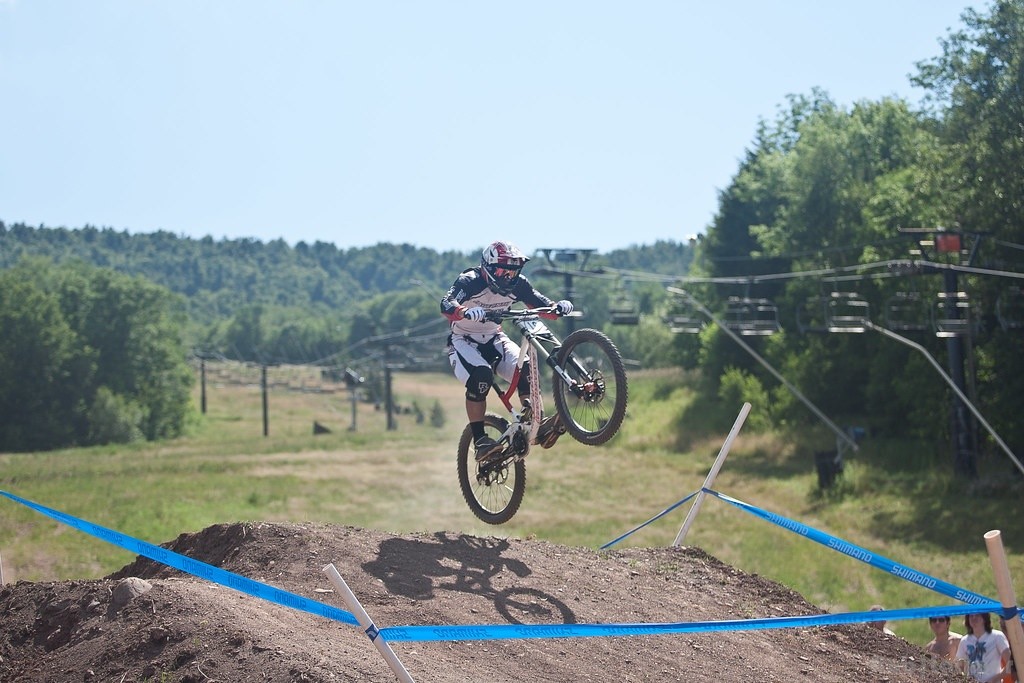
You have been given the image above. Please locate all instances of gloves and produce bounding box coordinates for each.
[555,300,573,316]
[464,307,485,322]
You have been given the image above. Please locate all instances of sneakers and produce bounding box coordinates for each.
[537,412,566,449]
[474,435,503,462]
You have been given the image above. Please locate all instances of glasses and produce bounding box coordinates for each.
[931,618,947,622]
[490,267,520,279]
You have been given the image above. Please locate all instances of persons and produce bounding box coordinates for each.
[924,613,964,669]
[439,240,575,460]
[863,604,896,637]
[955,612,1011,683]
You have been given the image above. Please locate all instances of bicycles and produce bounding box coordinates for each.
[457,305,629,526]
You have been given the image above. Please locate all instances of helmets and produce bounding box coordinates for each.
[480,241,530,296]
